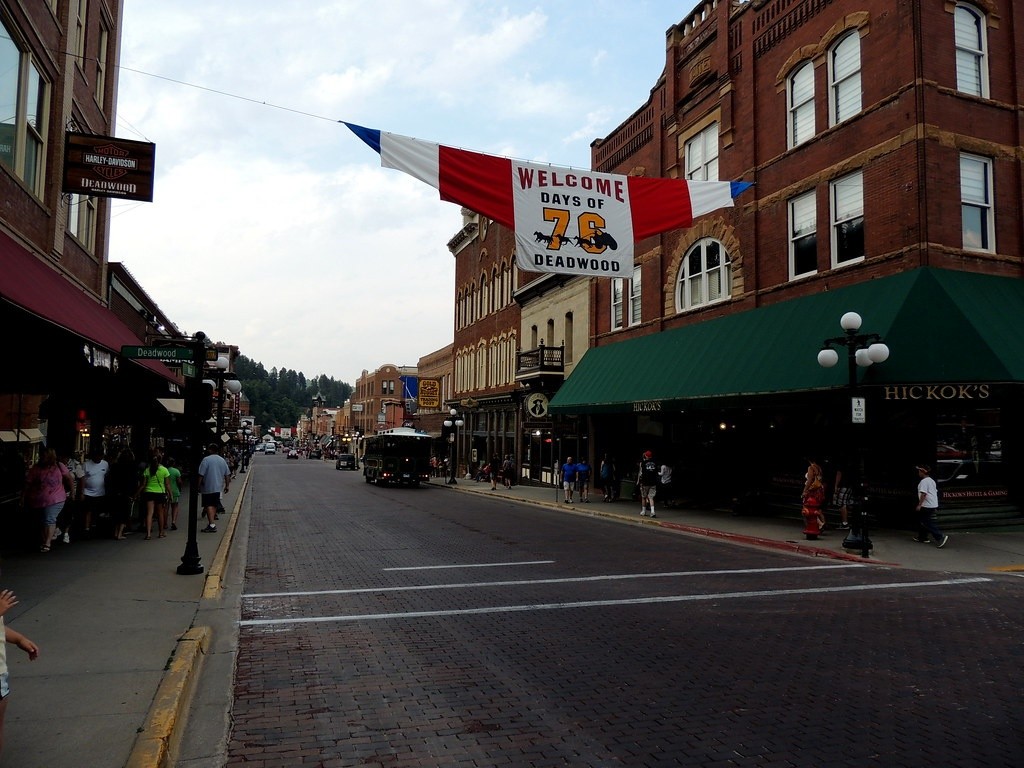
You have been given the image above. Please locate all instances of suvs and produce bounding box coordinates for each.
[336,454,355,471]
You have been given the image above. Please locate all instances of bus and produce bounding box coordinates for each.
[359,428,432,488]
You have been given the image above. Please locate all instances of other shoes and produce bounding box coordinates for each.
[602,496,616,503]
[39,542,51,552]
[564,498,574,504]
[143,535,152,540]
[159,532,167,538]
[580,496,589,503]
[491,487,496,491]
[504,484,511,490]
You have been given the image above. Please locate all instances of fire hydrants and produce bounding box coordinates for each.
[803,496,821,540]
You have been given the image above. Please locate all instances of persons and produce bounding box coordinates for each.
[576,457,592,503]
[0,588,38,755]
[429,456,451,478]
[912,465,948,547]
[21,442,237,553]
[473,452,516,490]
[560,456,578,503]
[600,451,622,502]
[800,455,826,532]
[636,450,677,517]
[833,461,853,530]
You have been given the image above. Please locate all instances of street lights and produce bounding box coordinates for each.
[818,312,890,556]
[202,357,242,515]
[444,409,463,485]
[237,422,251,473]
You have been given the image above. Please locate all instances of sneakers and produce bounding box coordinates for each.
[63,535,70,544]
[833,523,849,530]
[200,525,218,532]
[170,523,177,530]
[912,536,931,543]
[640,511,648,517]
[51,527,62,541]
[649,512,656,518]
[936,535,949,549]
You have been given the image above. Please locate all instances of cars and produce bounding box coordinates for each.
[255,441,322,460]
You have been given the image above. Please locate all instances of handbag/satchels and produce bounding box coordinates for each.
[62,478,71,493]
[161,495,169,506]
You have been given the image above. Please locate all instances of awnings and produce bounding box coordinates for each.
[544,265,1024,415]
[0,230,184,387]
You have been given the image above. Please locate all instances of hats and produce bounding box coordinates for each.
[915,464,931,475]
[645,451,652,459]
